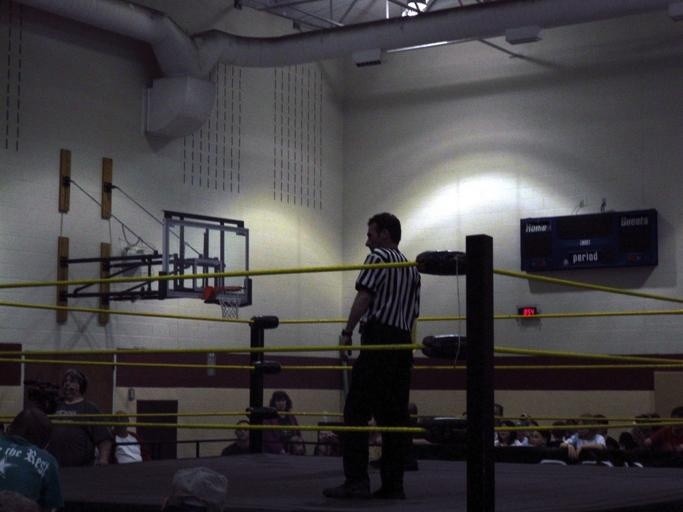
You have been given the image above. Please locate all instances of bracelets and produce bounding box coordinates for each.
[341,329,354,339]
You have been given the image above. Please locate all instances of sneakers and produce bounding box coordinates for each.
[366,484,408,501]
[322,480,373,500]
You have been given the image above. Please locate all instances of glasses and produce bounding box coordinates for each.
[64,367,81,378]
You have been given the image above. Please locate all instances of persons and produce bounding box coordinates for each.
[0,406,64,512]
[323,211,421,502]
[43,368,112,468]
[108,409,149,465]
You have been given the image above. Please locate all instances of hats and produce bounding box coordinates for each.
[169,466,230,504]
